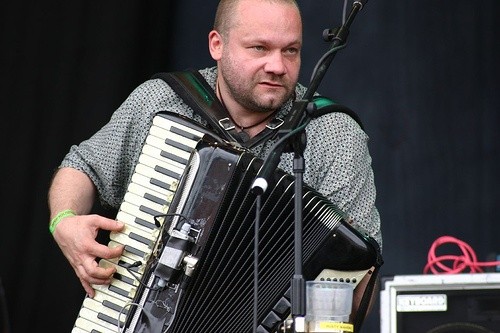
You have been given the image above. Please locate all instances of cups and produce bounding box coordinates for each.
[305,282,353,333]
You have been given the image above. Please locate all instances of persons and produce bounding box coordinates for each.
[47,0,384,333]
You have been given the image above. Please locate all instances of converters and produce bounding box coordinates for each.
[154,223,198,288]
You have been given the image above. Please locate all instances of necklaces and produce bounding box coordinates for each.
[215,81,276,142]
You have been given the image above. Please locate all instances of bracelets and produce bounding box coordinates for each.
[49,209,78,234]
